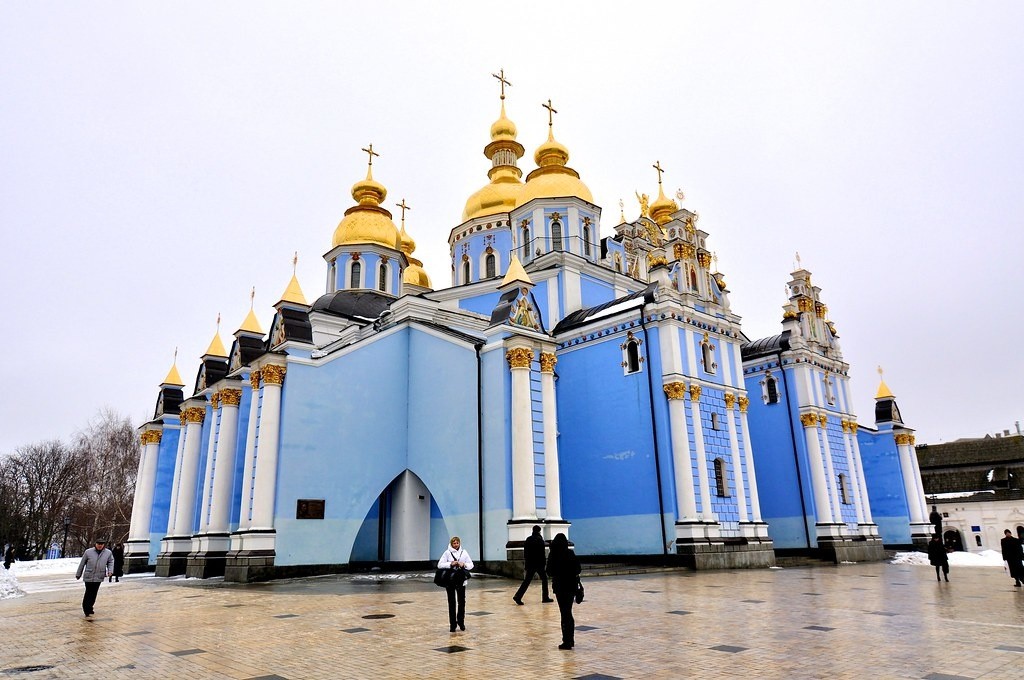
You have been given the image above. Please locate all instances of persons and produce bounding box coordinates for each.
[1001,529,1024,587]
[437,536,475,632]
[928,534,949,582]
[109,543,124,582]
[546,533,581,650]
[513,525,553,605]
[945,538,959,550]
[76,539,115,620]
[4,547,15,569]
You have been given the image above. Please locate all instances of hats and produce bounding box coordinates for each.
[96,538,106,544]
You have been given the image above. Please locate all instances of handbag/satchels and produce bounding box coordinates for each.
[434,567,462,590]
[942,564,949,573]
[576,586,584,604]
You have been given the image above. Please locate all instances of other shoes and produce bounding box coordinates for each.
[89,611,95,614]
[542,598,553,603]
[559,644,572,650]
[450,624,457,632]
[938,578,941,581]
[85,615,92,620]
[513,596,524,605]
[457,620,465,631]
[115,579,119,582]
[945,578,948,582]
[1014,583,1021,586]
[109,579,111,582]
